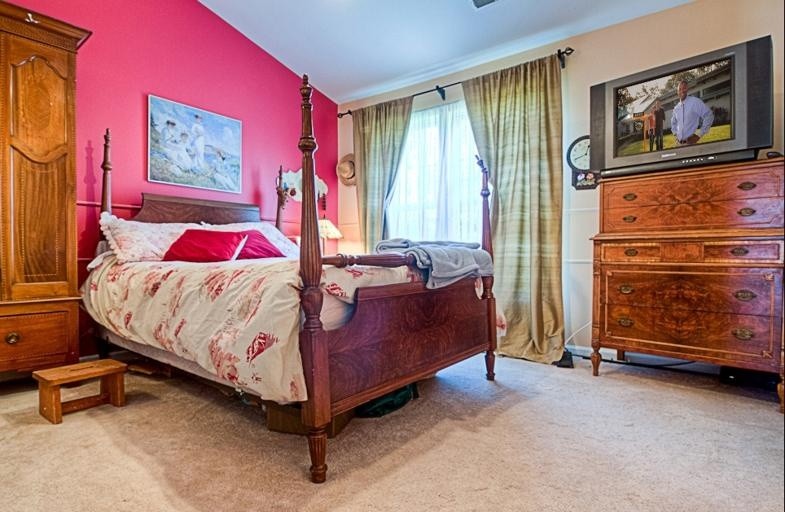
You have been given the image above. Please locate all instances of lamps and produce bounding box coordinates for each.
[318,218,343,257]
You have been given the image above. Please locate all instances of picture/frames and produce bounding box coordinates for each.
[147,94,242,194]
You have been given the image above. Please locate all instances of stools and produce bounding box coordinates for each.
[32,359,128,424]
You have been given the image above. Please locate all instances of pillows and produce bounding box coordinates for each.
[98,212,299,265]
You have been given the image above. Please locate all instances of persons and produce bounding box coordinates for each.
[157,109,239,194]
[670,80,715,146]
[647,104,657,152]
[654,97,667,151]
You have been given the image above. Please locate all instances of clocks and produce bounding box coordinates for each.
[567,136,598,190]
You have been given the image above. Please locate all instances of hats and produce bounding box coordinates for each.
[336,153,356,186]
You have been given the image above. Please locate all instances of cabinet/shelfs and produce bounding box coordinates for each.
[590,157,785,413]
[0,0,93,375]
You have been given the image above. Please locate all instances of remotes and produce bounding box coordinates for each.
[766,151,782,159]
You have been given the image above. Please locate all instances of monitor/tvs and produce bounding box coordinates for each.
[589,35,773,178]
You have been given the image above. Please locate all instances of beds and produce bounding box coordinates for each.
[98,74,497,483]
